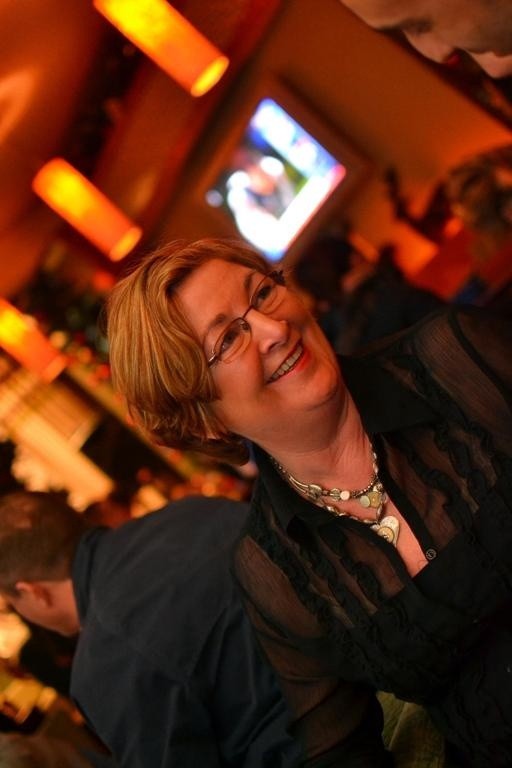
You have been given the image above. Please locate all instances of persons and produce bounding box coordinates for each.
[288,237,444,344]
[106,236,511,768]
[445,169,511,287]
[0,493,308,766]
[340,0,511,79]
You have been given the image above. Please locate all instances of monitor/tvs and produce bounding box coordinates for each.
[194,68,370,276]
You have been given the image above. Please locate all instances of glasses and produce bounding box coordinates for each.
[207,266,288,366]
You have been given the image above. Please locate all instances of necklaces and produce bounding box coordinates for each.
[268,442,400,548]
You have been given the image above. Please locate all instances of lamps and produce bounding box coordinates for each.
[0,298,70,387]
[93,0,230,99]
[31,156,143,264]
